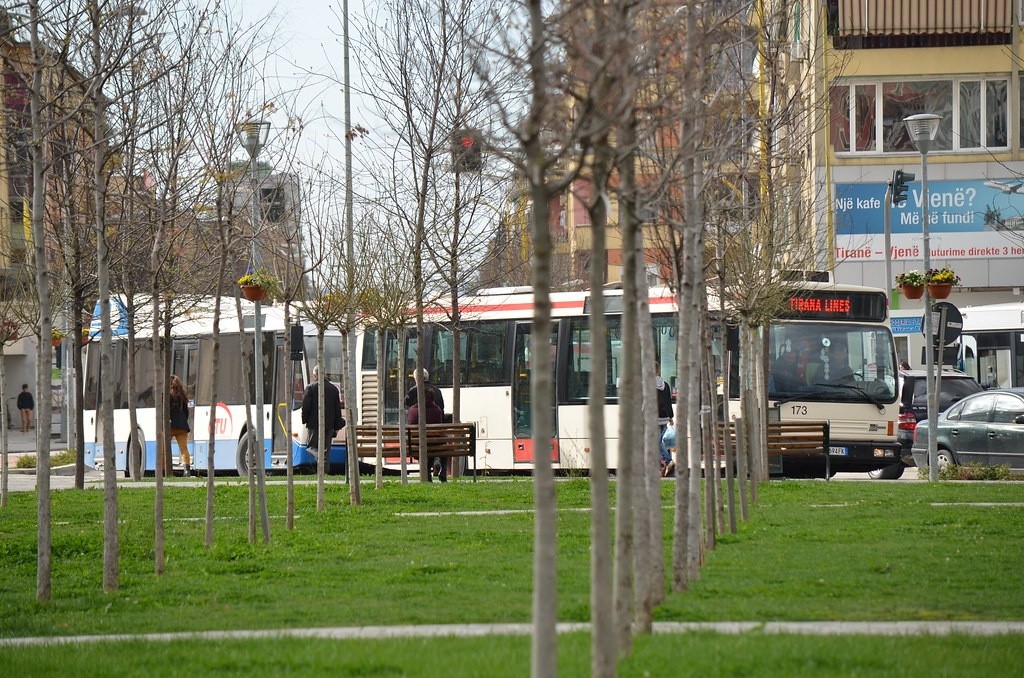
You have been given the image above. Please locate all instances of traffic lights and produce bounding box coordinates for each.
[894,170,914,203]
[451,127,483,174]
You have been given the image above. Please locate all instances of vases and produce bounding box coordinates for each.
[240,285,267,301]
[52,338,61,346]
[82,337,88,343]
[902,285,924,299]
[927,283,953,299]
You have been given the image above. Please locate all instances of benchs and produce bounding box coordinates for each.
[698,417,832,481]
[345,422,478,485]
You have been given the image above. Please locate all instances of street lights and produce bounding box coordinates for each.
[233,116,273,488]
[904,114,943,481]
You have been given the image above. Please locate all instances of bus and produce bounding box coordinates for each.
[77,290,348,483]
[353,270,900,479]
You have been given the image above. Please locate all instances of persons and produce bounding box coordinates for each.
[302,365,346,473]
[655,363,675,477]
[815,349,856,382]
[900,362,910,370]
[162,375,191,477]
[768,351,805,392]
[17,384,34,434]
[405,369,448,481]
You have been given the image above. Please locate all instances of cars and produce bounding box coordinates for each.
[900,366,1024,479]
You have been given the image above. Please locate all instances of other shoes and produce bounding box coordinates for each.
[428,473,432,482]
[439,473,447,483]
[664,460,674,477]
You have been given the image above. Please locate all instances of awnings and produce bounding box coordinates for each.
[838,0,1011,36]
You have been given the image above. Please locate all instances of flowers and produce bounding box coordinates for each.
[82,329,89,337]
[52,328,67,339]
[894,267,962,288]
[237,267,278,301]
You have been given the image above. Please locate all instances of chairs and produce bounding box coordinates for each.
[395,358,503,384]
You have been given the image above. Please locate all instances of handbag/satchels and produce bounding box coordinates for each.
[661,423,677,448]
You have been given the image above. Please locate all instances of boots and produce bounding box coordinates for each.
[183,464,190,478]
